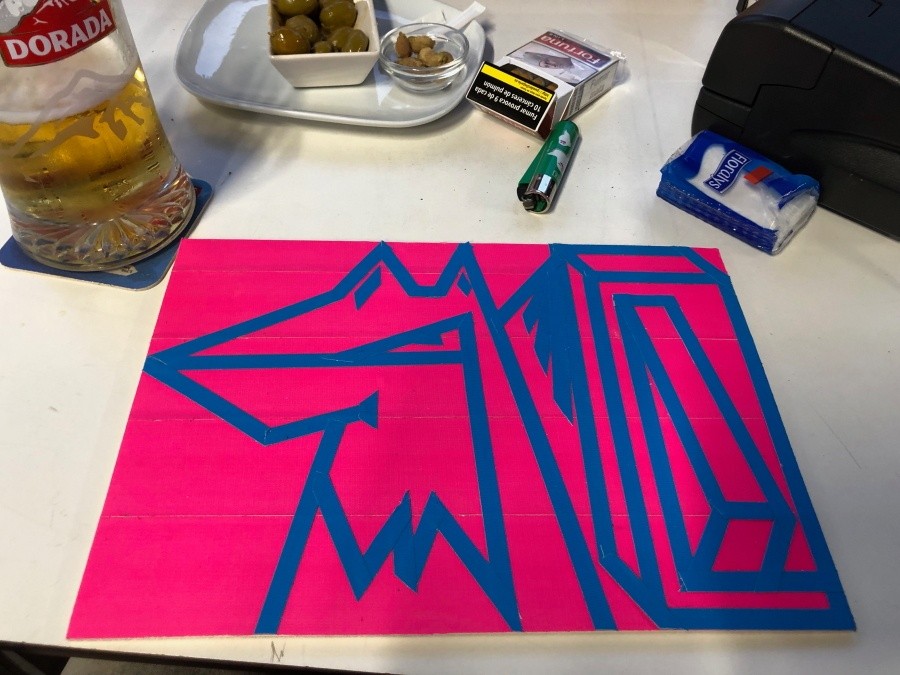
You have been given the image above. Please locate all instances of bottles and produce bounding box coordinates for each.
[1,0,197,273]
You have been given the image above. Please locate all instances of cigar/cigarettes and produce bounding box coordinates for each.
[509,67,555,93]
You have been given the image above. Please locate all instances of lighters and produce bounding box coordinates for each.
[516,118,580,213]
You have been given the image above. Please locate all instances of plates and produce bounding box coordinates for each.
[174,1,486,128]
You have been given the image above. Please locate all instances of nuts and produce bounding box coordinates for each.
[394,30,453,68]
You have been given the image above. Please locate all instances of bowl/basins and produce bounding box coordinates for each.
[267,0,381,88]
[380,24,470,96]
